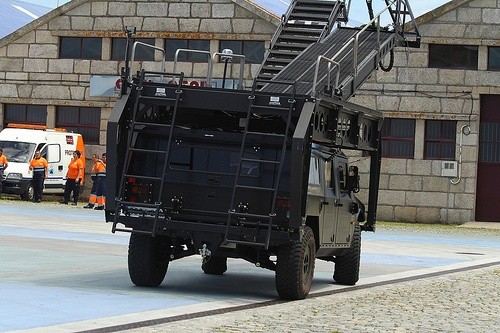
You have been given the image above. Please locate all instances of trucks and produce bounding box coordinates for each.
[105,42,384,300]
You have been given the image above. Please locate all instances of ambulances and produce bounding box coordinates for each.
[0,124,86,201]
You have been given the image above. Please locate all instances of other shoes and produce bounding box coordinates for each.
[60,201,68,205]
[71,203,77,206]
[94,206,103,210]
[84,204,93,208]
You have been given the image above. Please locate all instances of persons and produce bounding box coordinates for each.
[29,150,48,202]
[59,149,83,206]
[83,153,106,210]
[0,147,8,199]
